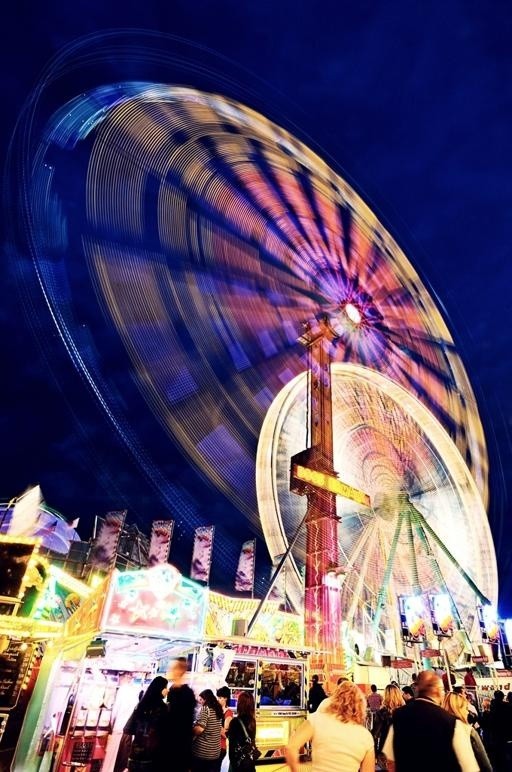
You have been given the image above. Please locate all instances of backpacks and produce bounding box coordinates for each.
[128,720,158,764]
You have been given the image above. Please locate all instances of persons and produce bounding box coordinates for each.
[367,666,512,772]
[258,662,328,712]
[122,657,257,772]
[285,681,376,772]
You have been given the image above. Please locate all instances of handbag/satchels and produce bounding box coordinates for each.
[235,737,263,767]
